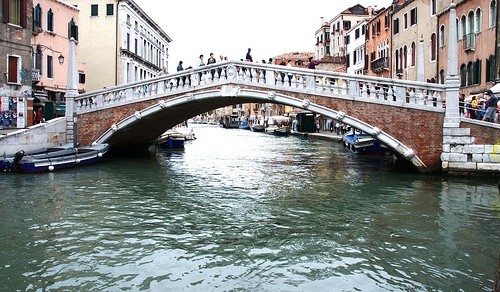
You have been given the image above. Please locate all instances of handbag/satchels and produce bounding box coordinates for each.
[466,104,472,112]
[245,59,251,62]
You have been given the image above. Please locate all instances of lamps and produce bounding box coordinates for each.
[31,44,65,65]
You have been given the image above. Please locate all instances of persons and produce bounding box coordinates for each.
[317,123,320,132]
[427,78,437,106]
[459,89,500,124]
[240,48,321,85]
[158,66,173,85]
[330,121,346,135]
[177,61,192,86]
[200,53,228,80]
[0,96,43,128]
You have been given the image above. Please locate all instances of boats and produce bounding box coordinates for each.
[342,127,382,153]
[157,126,195,151]
[221,115,293,136]
[0,142,111,174]
